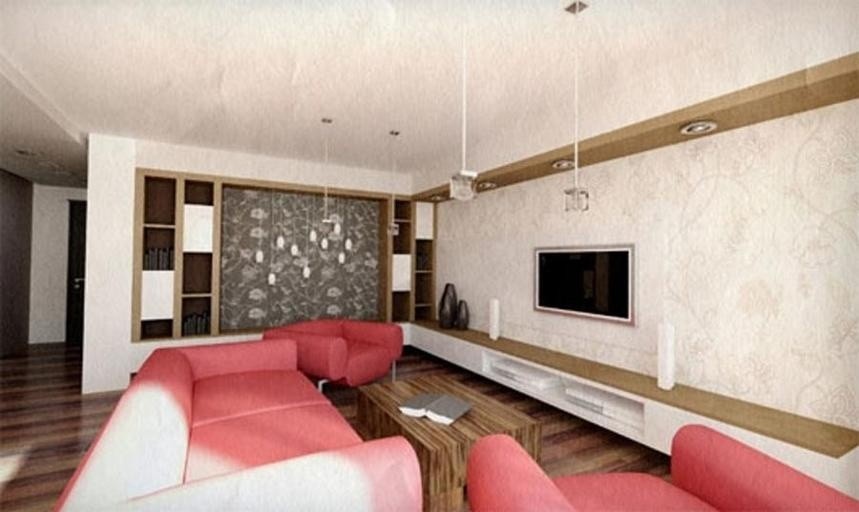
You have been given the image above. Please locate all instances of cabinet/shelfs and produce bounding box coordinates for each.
[132,169,223,344]
[380,196,439,325]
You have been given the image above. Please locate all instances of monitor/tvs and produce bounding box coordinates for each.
[533,243,637,325]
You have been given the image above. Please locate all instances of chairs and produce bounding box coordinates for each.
[465,423,859,512]
[262,319,404,388]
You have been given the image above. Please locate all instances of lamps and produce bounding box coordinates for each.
[450,20,482,201]
[243,116,359,288]
[557,18,590,214]
[382,132,405,237]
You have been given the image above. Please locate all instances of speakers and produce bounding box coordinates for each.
[489,299,500,341]
[656,321,676,390]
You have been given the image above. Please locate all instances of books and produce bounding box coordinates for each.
[397,391,473,428]
[183,311,209,336]
[143,246,174,271]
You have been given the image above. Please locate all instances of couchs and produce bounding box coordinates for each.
[49,340,424,512]
